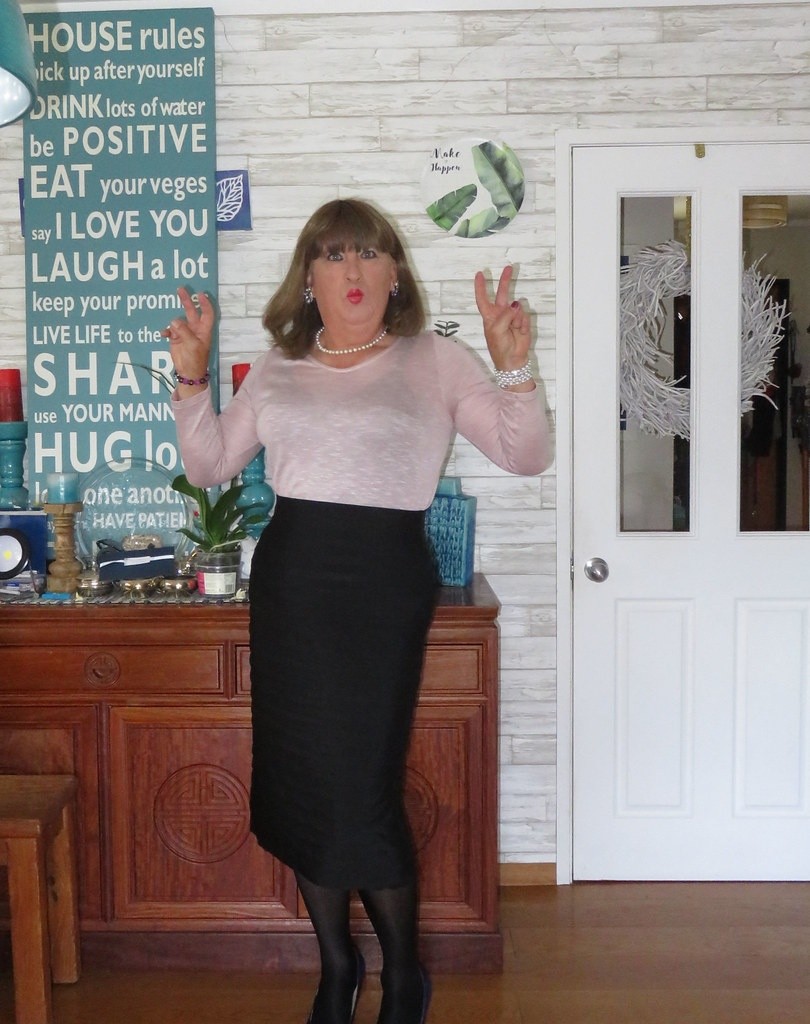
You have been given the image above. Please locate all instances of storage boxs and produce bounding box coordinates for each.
[0,511,48,575]
[429,495,478,588]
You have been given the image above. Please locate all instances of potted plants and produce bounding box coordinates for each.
[169,474,270,598]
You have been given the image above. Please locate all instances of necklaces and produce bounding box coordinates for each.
[316,326,389,354]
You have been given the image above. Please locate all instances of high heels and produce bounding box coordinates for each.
[305,942,365,1024]
[377,961,430,1024]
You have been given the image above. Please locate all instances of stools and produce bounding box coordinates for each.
[0,774,82,1024]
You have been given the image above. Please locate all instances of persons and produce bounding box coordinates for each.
[163,199,556,1023]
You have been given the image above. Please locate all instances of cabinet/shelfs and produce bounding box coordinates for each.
[0,572,507,975]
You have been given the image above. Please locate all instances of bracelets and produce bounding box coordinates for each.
[493,359,533,389]
[174,369,211,385]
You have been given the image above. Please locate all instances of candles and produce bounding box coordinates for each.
[0,369,25,422]
[231,362,251,398]
[47,472,80,503]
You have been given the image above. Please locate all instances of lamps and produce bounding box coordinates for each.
[0,0,38,129]
